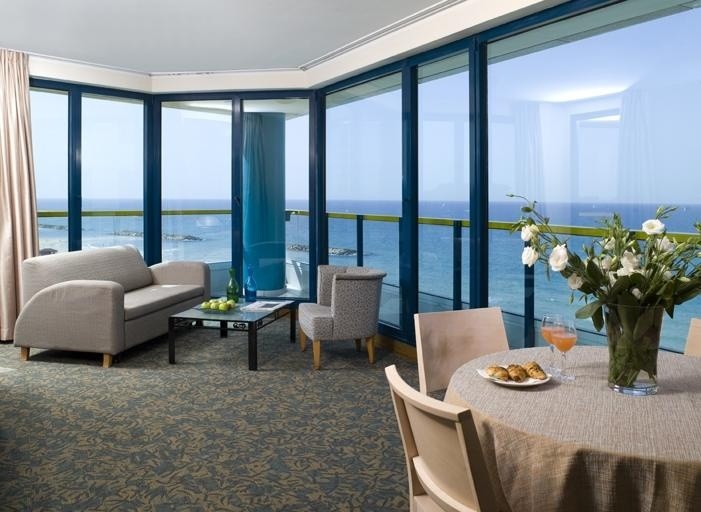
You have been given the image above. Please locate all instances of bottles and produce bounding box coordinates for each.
[244,263,258,301]
[227,268,241,302]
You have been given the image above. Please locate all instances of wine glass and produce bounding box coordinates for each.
[542,316,579,381]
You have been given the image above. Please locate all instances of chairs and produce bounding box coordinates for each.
[413,307,510,396]
[385,365,498,512]
[684,317,701,359]
[298,264,386,370]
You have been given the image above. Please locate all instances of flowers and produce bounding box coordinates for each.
[505,194,701,387]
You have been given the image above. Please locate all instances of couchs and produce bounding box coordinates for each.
[14,245,210,368]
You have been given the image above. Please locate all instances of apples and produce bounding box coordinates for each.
[201,299,235,311]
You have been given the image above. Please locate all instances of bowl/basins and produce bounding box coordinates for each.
[190,305,242,314]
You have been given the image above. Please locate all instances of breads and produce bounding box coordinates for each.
[488,361,547,382]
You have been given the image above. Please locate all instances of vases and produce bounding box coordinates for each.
[227,269,239,304]
[244,267,256,302]
[603,304,665,395]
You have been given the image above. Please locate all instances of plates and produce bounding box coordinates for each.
[477,368,553,387]
